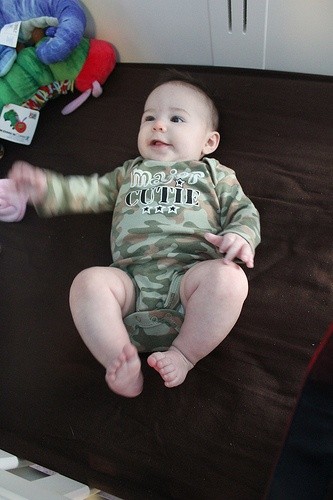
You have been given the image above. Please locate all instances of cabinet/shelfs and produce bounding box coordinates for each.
[75,1,332,81]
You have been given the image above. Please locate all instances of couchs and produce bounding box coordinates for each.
[0,62,333,500]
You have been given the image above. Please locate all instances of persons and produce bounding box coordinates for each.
[8,81,261,398]
